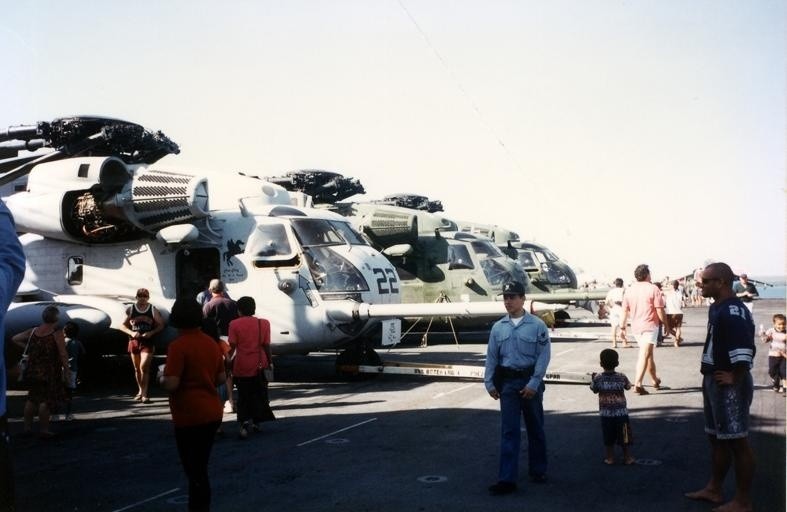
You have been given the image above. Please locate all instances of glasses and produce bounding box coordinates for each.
[702,277,721,283]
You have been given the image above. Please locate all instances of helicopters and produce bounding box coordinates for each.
[0,116,567,399]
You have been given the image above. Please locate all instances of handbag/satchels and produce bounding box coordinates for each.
[16,354,30,381]
[259,366,274,382]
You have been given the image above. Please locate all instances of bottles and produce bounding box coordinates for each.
[758,323,768,343]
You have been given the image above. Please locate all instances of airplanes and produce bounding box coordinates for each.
[668,260,773,307]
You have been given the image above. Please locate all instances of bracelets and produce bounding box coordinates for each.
[620,326,625,330]
[158,374,165,378]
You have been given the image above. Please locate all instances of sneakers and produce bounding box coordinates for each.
[239,420,249,438]
[772,381,780,391]
[675,337,683,347]
[489,480,517,496]
[653,377,662,390]
[51,414,59,422]
[634,386,649,393]
[533,472,547,482]
[65,414,75,421]
[782,391,787,397]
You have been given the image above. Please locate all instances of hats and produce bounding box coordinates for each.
[498,281,525,296]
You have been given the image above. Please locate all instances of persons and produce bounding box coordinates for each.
[583,274,759,347]
[157,297,232,511]
[0,201,25,509]
[12,304,69,438]
[758,314,785,393]
[617,266,668,393]
[590,348,636,465]
[193,278,276,438]
[49,322,81,421]
[120,288,165,401]
[683,262,754,511]
[484,281,550,493]
